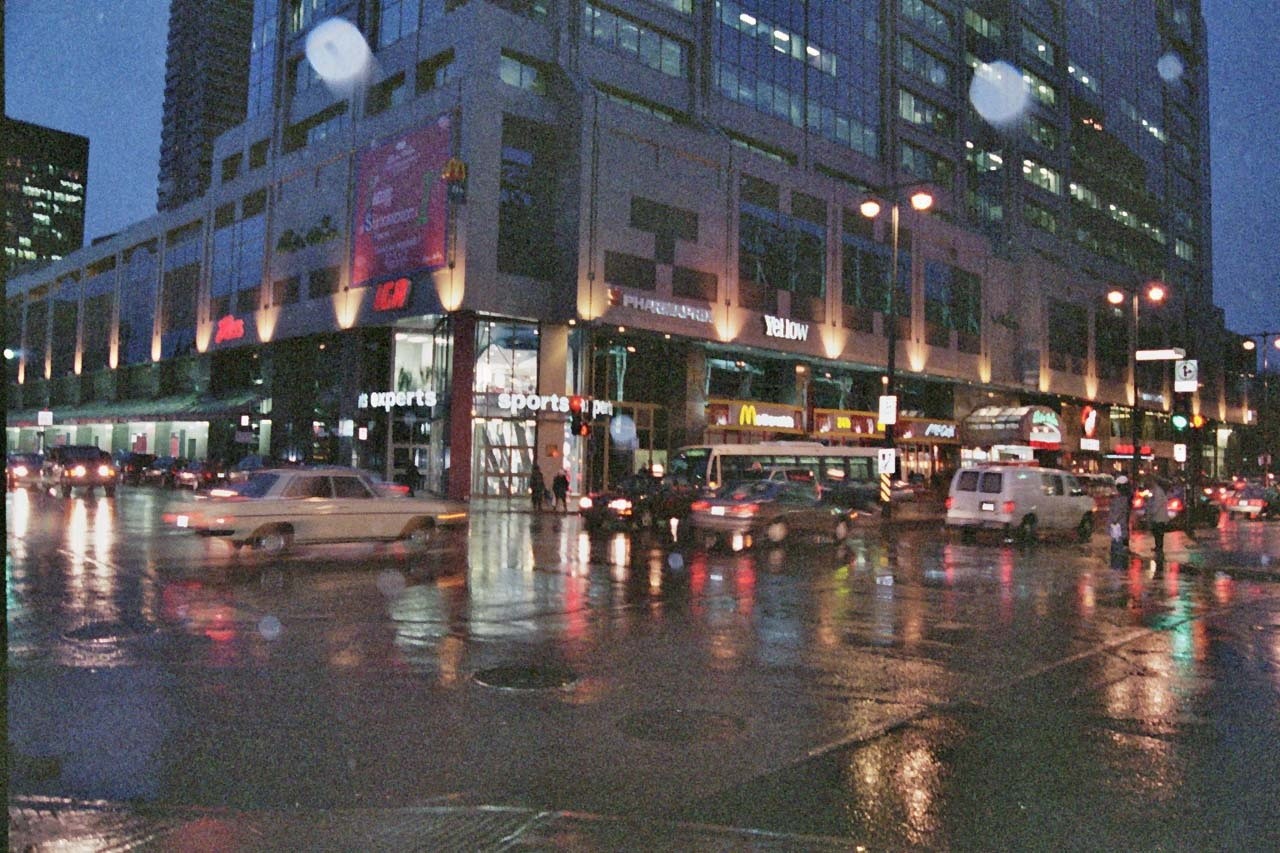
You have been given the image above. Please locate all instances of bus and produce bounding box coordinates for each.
[662,441,881,503]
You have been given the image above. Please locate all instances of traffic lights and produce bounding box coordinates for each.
[569,398,589,436]
[1172,393,1192,434]
[1193,414,1205,437]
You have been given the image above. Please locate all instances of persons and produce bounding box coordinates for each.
[529,465,546,511]
[1112,469,1167,553]
[406,460,420,498]
[551,469,570,513]
[637,462,648,474]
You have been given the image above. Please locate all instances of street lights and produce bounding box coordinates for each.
[1108,282,1166,490]
[1243,332,1280,487]
[861,182,931,514]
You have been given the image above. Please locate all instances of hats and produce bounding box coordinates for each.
[1152,475,1162,485]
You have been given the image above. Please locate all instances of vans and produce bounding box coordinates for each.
[41,444,117,496]
[946,465,1097,540]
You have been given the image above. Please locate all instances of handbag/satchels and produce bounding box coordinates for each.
[1109,522,1122,542]
[544,489,552,499]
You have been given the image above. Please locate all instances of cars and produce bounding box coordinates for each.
[4,450,46,491]
[891,478,914,509]
[116,452,279,490]
[1079,473,1280,529]
[161,465,468,561]
[690,482,854,545]
[577,476,692,530]
